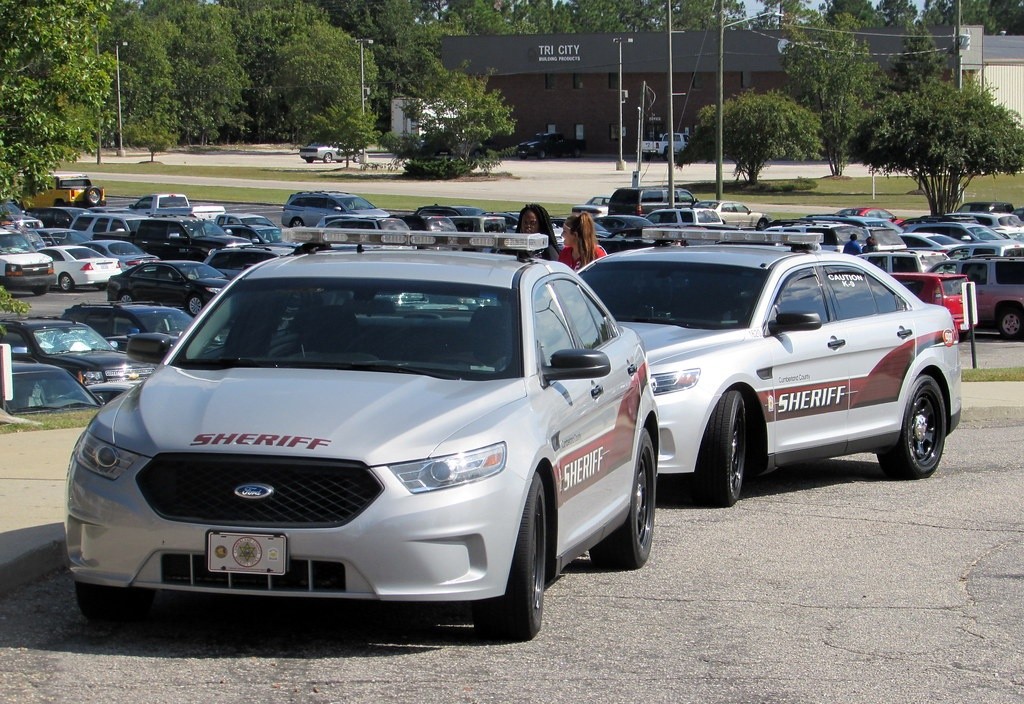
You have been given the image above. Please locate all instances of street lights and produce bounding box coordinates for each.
[714,1,787,199]
[115,42,130,150]
[357,39,376,115]
[614,36,634,164]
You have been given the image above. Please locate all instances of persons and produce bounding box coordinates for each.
[862,237,873,253]
[843,234,862,255]
[516,204,560,260]
[558,212,608,271]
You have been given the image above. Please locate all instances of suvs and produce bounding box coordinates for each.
[61,300,196,352]
[1,316,158,403]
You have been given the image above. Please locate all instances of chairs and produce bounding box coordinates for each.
[458,303,513,368]
[284,290,375,356]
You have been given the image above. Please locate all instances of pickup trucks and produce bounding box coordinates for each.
[518,133,586,160]
[641,132,691,162]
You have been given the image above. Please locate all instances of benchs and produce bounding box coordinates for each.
[355,312,473,363]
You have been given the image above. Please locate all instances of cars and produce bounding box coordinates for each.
[574,228,964,508]
[65,227,661,641]
[4,360,105,416]
[299,140,364,164]
[0,174,1024,344]
